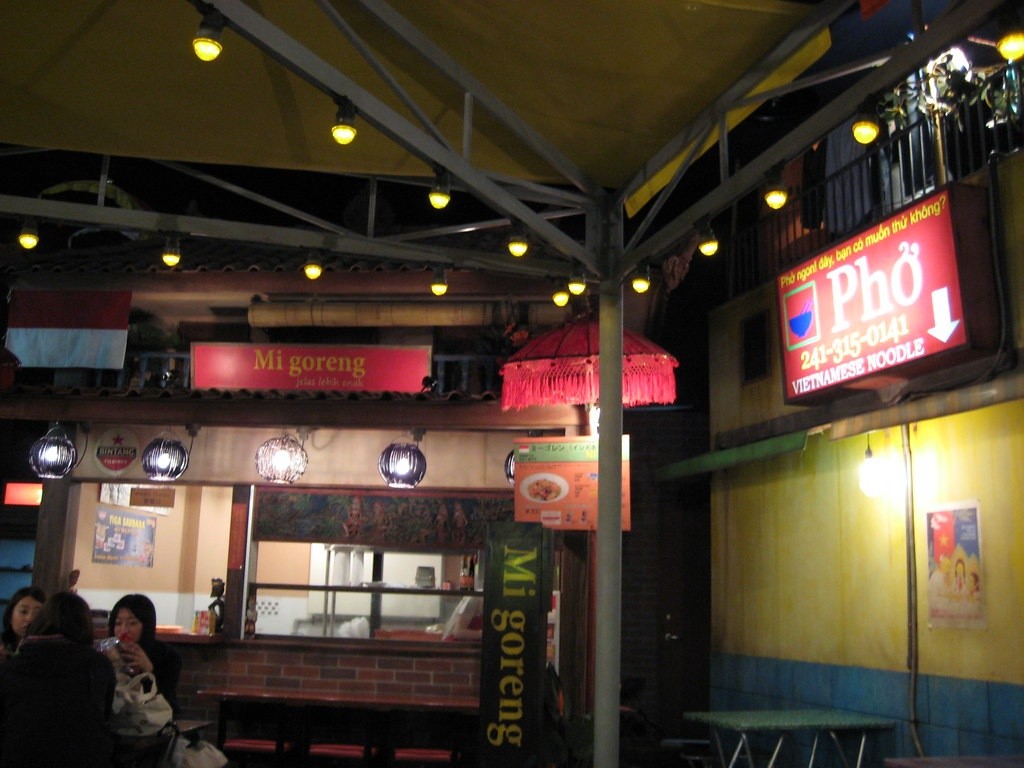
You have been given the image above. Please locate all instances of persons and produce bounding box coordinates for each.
[0,586,180,768]
[951,559,980,600]
[443,586,483,643]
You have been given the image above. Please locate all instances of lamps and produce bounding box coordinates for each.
[549,261,587,307]
[331,97,357,146]
[694,213,719,255]
[989,0,1024,61]
[191,2,229,62]
[378,427,427,488]
[27,421,77,479]
[428,163,529,303]
[141,424,189,482]
[504,429,542,487]
[633,256,653,294]
[852,91,880,144]
[763,158,787,211]
[16,215,325,279]
[255,426,308,484]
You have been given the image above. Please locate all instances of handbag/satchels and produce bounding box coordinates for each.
[109,671,174,738]
[165,734,229,768]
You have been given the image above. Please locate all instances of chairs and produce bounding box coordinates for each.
[214,698,463,768]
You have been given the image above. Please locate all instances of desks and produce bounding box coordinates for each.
[682,708,895,768]
[116,719,214,768]
[198,686,479,768]
